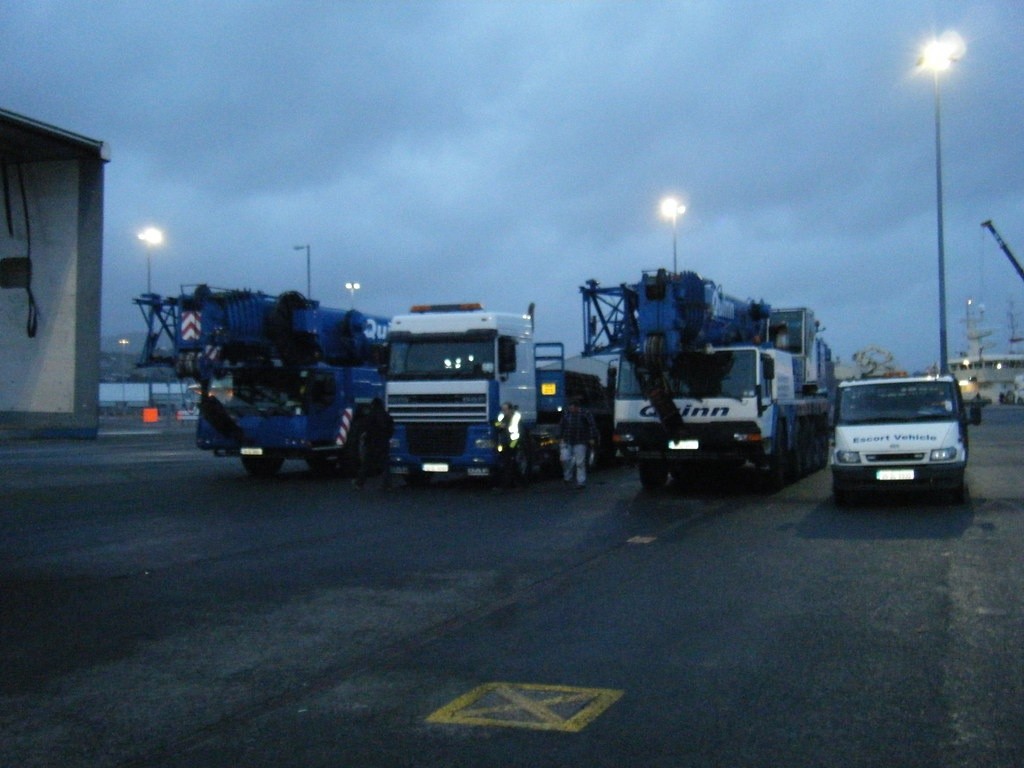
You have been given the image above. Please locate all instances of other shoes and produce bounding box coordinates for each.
[516,487,527,493]
[576,485,586,489]
[567,481,575,494]
[493,488,505,494]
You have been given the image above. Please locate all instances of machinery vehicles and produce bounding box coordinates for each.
[575,267,837,500]
[131,283,391,486]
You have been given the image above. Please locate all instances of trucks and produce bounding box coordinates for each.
[823,375,981,506]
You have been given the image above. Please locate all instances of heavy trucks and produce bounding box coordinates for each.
[385,302,617,494]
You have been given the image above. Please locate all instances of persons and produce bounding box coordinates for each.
[493,402,531,489]
[558,398,597,496]
[352,398,394,494]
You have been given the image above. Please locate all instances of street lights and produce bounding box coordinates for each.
[139,226,161,294]
[918,34,950,376]
[294,244,310,301]
[661,195,684,276]
